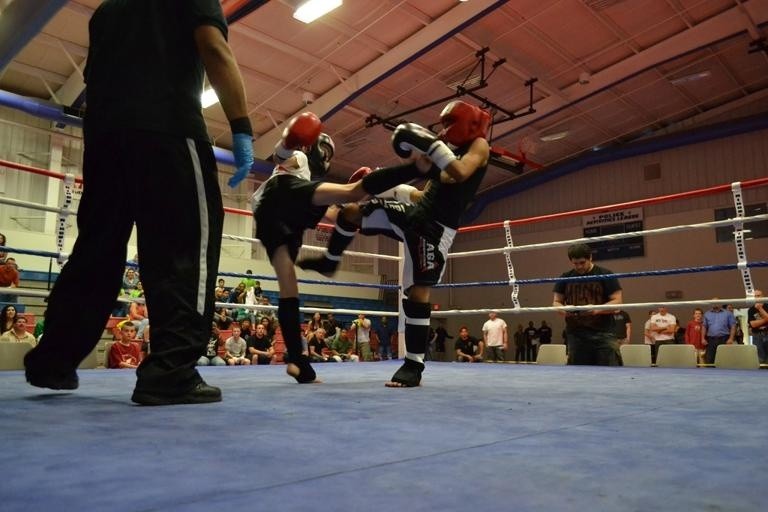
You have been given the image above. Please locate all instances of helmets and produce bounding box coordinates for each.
[438,100,493,146]
[301,131,336,178]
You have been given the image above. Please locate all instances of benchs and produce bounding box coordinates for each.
[217,288,398,365]
[0,266,125,347]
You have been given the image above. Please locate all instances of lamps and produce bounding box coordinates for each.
[292,0,343,24]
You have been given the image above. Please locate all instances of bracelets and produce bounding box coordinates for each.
[228,116,253,137]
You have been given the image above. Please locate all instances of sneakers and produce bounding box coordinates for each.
[24,371,80,391]
[132,379,222,406]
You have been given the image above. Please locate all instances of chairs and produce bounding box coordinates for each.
[78,345,99,368]
[102,342,122,369]
[619,344,652,367]
[0,342,34,370]
[536,344,570,366]
[655,344,697,368]
[714,344,760,370]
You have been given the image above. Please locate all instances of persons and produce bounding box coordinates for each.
[254,112,434,383]
[198,269,278,366]
[612,289,768,369]
[23,0,254,408]
[296,100,493,387]
[0,233,48,348]
[427,311,553,364]
[106,252,151,370]
[551,244,623,366]
[298,311,398,364]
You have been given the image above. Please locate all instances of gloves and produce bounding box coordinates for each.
[226,133,256,188]
